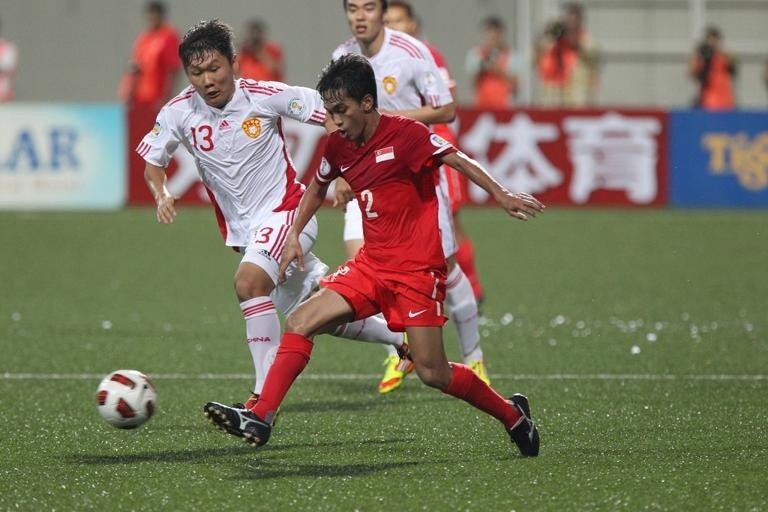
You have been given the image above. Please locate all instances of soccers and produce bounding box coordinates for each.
[95,368,154,429]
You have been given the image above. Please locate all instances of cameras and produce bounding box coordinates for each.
[548,19,566,41]
[696,41,714,61]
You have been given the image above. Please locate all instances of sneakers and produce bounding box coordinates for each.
[204,395,278,445]
[378,332,414,394]
[510,394,539,458]
[471,360,491,388]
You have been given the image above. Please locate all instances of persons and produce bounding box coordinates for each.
[379,1,485,304]
[202,52,547,456]
[533,3,599,109]
[119,2,188,106]
[329,1,490,393]
[134,15,414,429]
[463,14,521,108]
[686,22,746,108]
[229,17,290,83]
